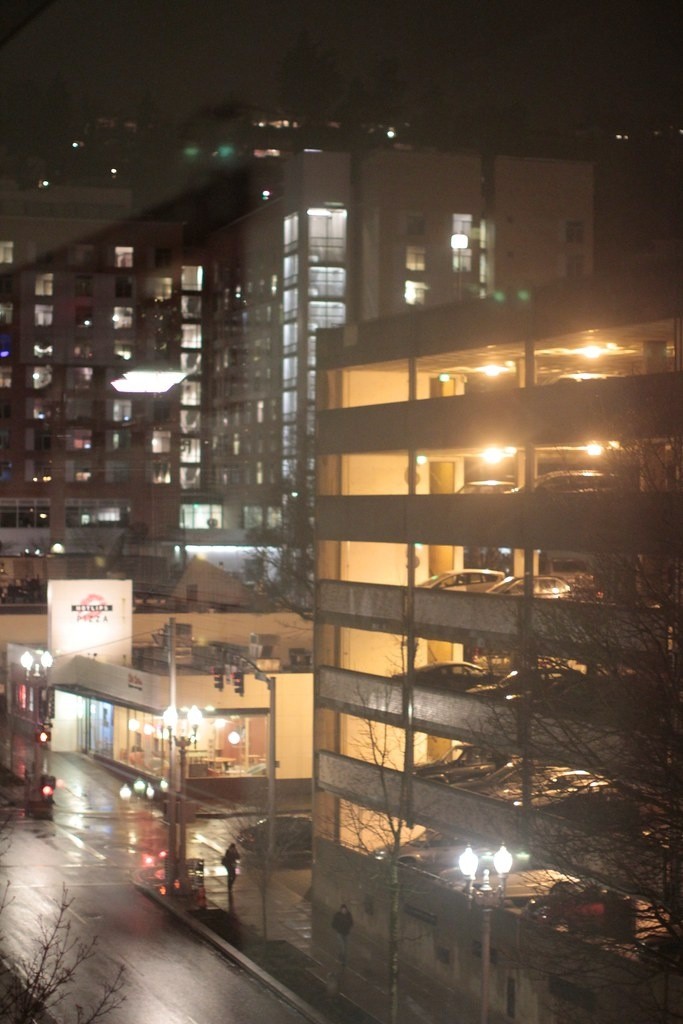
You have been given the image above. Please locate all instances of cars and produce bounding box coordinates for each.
[505,672,605,719]
[438,849,584,905]
[370,826,535,870]
[457,480,517,495]
[520,879,640,937]
[416,568,506,598]
[237,814,314,852]
[513,467,622,496]
[511,769,615,813]
[391,660,493,695]
[464,660,585,699]
[449,760,572,801]
[484,575,574,602]
[409,739,511,784]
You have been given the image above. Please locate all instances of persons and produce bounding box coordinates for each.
[223,843,240,889]
[332,905,353,966]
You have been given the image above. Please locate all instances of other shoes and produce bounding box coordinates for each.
[231,881,234,884]
[228,886,232,889]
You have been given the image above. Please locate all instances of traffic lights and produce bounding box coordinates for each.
[211,665,225,689]
[41,775,56,806]
[38,731,52,743]
[232,671,244,696]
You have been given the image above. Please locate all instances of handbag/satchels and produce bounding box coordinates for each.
[221,857,226,866]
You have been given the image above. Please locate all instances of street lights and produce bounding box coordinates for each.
[459,841,513,1024]
[164,704,203,900]
[15,651,54,796]
